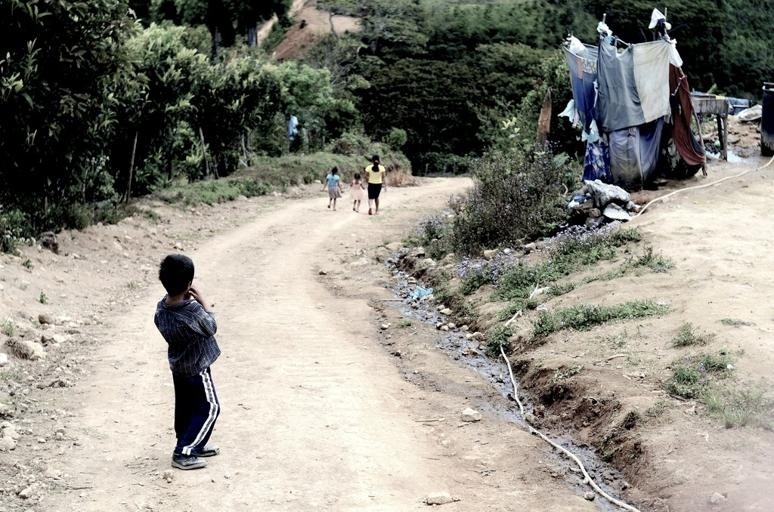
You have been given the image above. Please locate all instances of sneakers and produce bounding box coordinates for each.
[171,447,221,470]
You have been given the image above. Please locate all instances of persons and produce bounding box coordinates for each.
[287,110,298,153]
[321,167,343,211]
[295,122,309,152]
[348,173,364,212]
[364,154,385,215]
[153,253,221,469]
